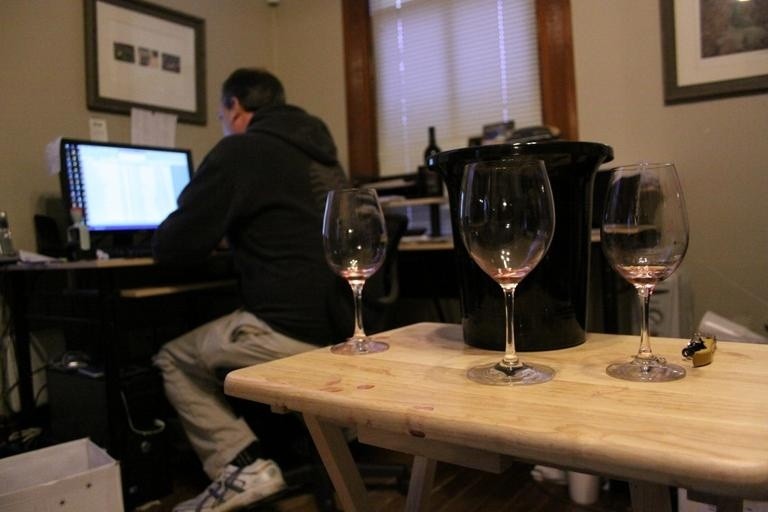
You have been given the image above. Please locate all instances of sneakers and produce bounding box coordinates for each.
[171,456,288,512]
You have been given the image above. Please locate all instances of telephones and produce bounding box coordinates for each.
[0,211,21,263]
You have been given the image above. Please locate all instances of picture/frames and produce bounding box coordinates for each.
[655,0,768,105]
[84,1,210,126]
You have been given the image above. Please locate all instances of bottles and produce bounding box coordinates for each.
[423,123,446,196]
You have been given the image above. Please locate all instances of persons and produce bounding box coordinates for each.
[147,67,352,512]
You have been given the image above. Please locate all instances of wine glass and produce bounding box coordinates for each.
[601,161,689,384]
[462,158,558,386]
[323,188,391,355]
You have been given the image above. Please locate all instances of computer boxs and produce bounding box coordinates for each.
[46,344,174,509]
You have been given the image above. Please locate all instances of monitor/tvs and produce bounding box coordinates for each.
[59,135,193,258]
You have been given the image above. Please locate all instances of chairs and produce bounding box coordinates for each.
[213,212,413,510]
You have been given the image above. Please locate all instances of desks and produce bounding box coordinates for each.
[222,319,768,512]
[3,196,621,447]
